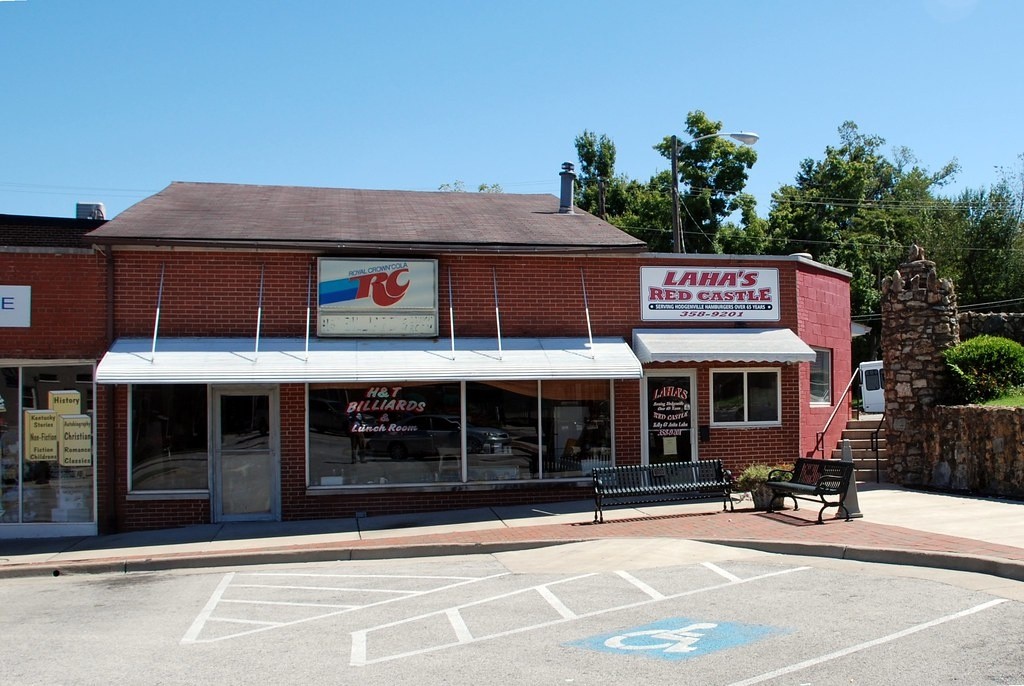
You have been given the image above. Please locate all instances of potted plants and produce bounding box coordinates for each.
[736,463,794,509]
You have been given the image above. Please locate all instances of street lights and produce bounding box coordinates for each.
[666,128,760,257]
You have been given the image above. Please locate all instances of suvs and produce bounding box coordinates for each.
[365,413,489,459]
[439,415,512,446]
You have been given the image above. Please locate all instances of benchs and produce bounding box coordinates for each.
[764,458,854,525]
[592,459,734,523]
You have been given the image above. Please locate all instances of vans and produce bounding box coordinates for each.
[861,360,886,413]
